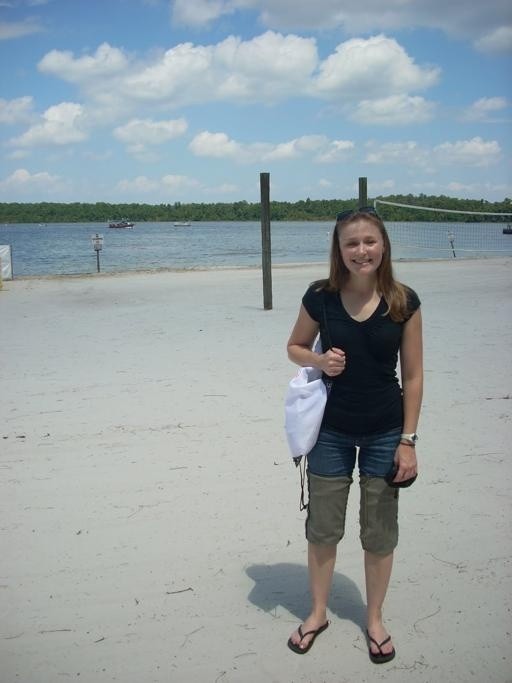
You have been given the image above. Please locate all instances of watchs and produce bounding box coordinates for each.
[400,433,418,443]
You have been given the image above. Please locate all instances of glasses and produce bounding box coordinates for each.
[334,203,379,218]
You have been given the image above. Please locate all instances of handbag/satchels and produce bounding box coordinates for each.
[281,340,335,469]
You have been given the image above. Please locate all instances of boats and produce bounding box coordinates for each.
[173,220,193,227]
[107,219,135,229]
[502,223,512,234]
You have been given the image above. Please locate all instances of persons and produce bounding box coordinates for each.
[287,207,424,664]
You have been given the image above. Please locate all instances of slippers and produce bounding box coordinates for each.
[365,626,398,665]
[287,620,331,657]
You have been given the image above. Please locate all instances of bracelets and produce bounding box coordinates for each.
[399,441,415,446]
